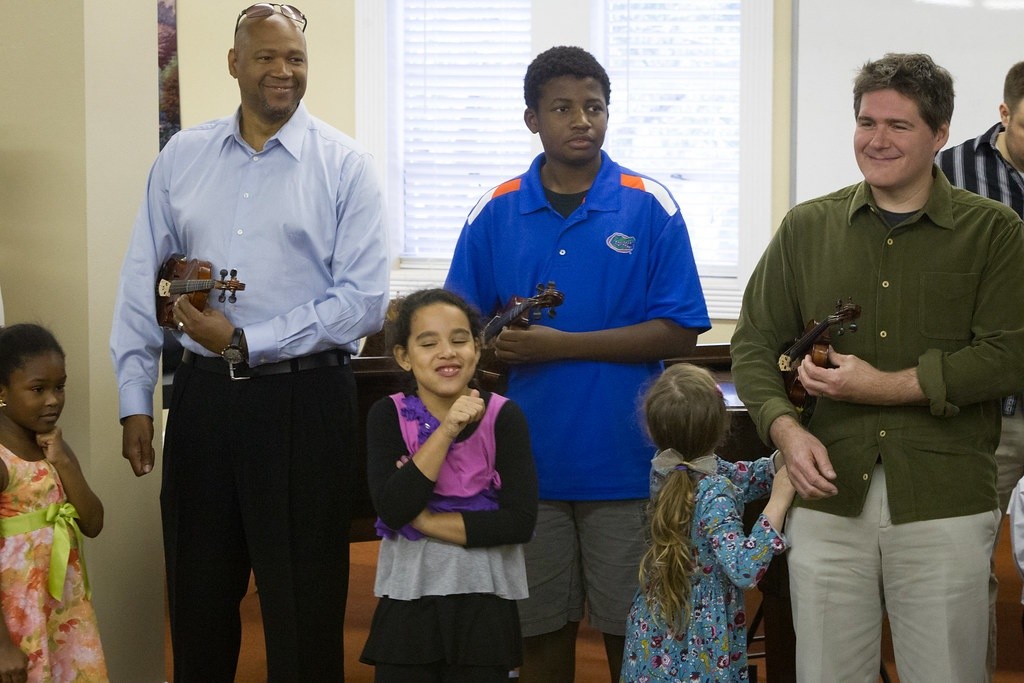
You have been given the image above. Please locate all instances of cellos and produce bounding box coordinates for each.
[877,491,1024,683]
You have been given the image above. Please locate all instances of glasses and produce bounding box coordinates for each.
[236,3,309,35]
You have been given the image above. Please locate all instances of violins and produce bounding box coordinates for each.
[777,296,862,408]
[154,252,246,330]
[473,280,565,390]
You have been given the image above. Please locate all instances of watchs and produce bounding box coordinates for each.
[220,326,247,365]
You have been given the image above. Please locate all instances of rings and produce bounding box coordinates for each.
[176,321,186,330]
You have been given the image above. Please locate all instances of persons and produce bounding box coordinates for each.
[618,363,798,683]
[0,321,111,683]
[359,288,536,683]
[730,54,1024,683]
[111,3,387,683]
[442,46,711,683]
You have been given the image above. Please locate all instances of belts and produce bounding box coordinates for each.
[182,347,352,382]
